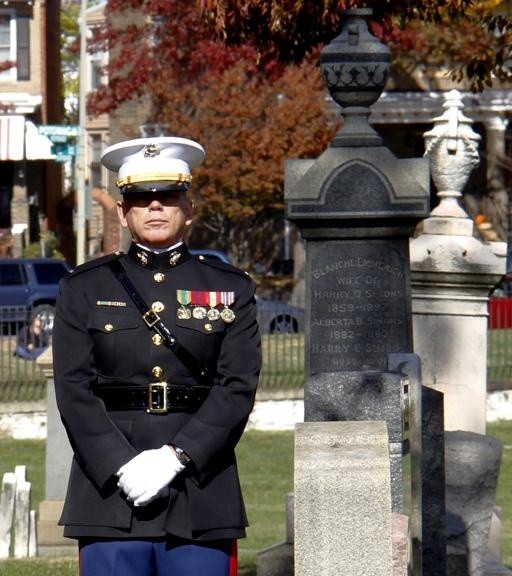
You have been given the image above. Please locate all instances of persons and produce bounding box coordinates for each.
[16,317,49,361]
[53,136,263,576]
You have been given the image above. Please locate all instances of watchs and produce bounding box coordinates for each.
[170,445,191,465]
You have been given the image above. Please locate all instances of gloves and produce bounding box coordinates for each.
[115,444,186,507]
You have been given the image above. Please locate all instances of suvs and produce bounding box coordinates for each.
[0,257,76,338]
[187,251,305,336]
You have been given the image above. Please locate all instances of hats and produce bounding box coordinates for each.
[100,136,206,193]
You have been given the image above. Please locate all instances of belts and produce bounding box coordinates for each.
[96,382,212,413]
